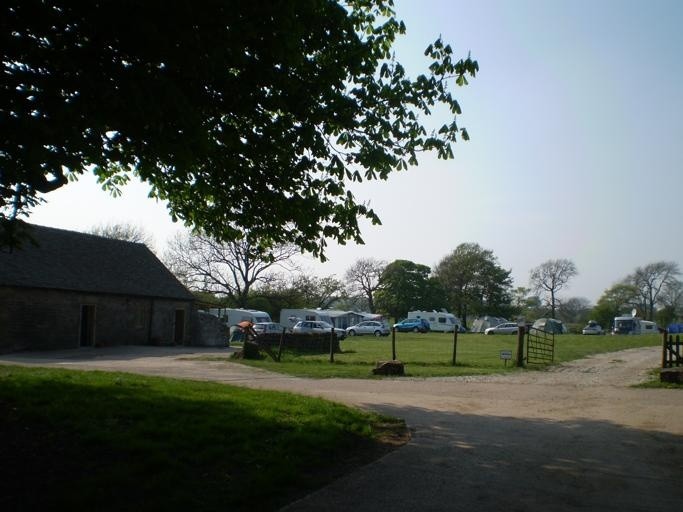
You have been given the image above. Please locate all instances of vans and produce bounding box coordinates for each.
[209,308,271,340]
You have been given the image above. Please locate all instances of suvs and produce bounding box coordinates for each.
[292,321,345,340]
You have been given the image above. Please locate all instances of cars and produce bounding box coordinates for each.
[248,322,290,342]
[484,323,527,335]
[392,318,430,332]
[346,321,389,337]
